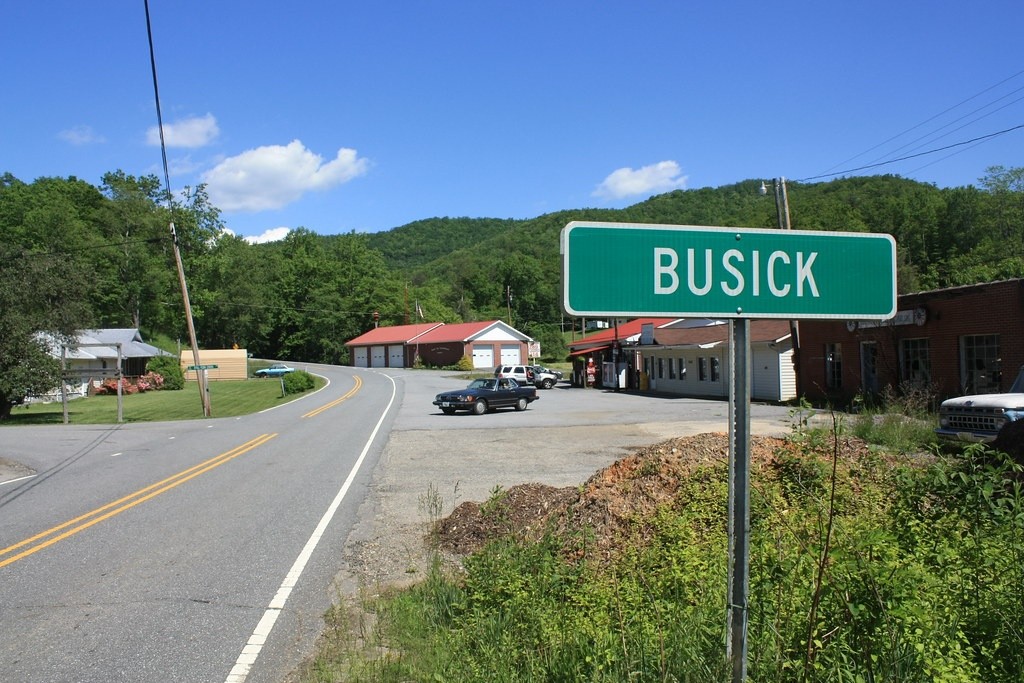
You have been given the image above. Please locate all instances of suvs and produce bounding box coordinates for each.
[493,364,557,389]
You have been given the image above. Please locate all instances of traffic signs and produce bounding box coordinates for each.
[187,364,218,371]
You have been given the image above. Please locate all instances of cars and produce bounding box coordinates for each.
[530,365,563,379]
[253,363,295,379]
[432,378,538,415]
[933,364,1024,462]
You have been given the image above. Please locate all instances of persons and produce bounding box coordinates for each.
[587,362,596,388]
[527,369,535,381]
